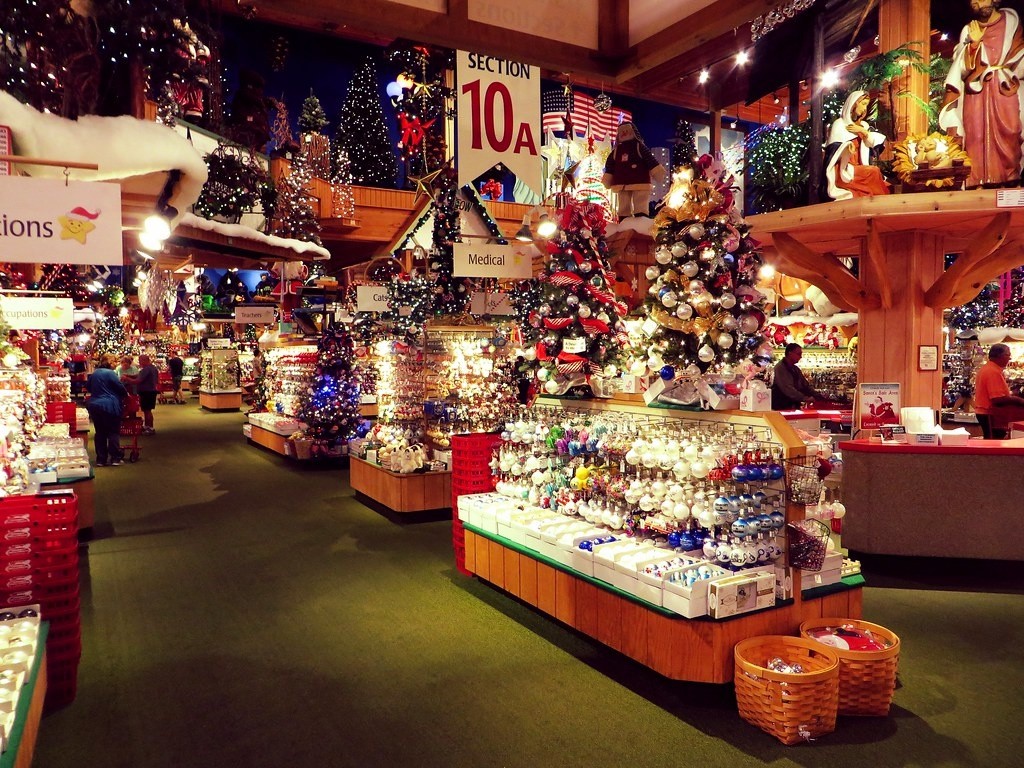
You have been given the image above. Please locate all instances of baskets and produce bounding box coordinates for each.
[734,638,840,746]
[47,402,77,439]
[799,618,899,718]
[779,454,826,508]
[0,488,80,717]
[787,517,832,571]
[120,417,143,436]
[451,432,504,579]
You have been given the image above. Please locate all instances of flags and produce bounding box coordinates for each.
[542,90,632,142]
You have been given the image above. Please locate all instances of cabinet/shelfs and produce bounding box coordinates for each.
[469,400,862,681]
[199,325,492,512]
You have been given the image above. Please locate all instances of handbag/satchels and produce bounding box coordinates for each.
[122,394,140,418]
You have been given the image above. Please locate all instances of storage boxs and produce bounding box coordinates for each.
[458,493,792,618]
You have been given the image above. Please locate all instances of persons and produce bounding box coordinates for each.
[167,350,185,405]
[115,355,158,435]
[939,0,1024,190]
[253,348,262,382]
[819,90,890,202]
[87,353,128,465]
[771,343,829,411]
[975,344,1012,440]
[915,137,952,168]
[602,122,666,224]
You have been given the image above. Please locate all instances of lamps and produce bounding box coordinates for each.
[139,206,178,259]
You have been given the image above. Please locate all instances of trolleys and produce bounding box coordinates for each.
[805,397,854,433]
[109,393,146,463]
[156,370,179,405]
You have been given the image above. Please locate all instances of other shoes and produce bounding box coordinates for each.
[111,459,124,465]
[141,426,155,435]
[180,400,186,405]
[97,461,109,466]
